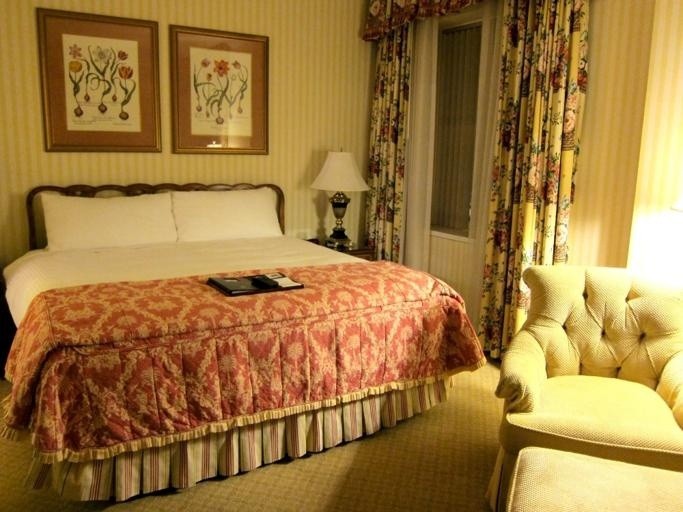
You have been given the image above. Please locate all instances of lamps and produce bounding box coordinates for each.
[310,147,371,249]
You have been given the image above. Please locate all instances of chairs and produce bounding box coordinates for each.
[486,265,683,511]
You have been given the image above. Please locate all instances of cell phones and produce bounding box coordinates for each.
[253,274,279,289]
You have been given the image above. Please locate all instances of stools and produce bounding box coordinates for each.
[505,447,683,512]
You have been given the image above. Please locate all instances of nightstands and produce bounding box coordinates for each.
[307,238,377,262]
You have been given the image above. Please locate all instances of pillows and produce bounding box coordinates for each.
[172,187,284,241]
[40,192,176,250]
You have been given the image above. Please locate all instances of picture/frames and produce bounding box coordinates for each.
[168,24,269,155]
[35,6,161,152]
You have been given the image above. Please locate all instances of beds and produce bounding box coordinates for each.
[2,183,487,502]
